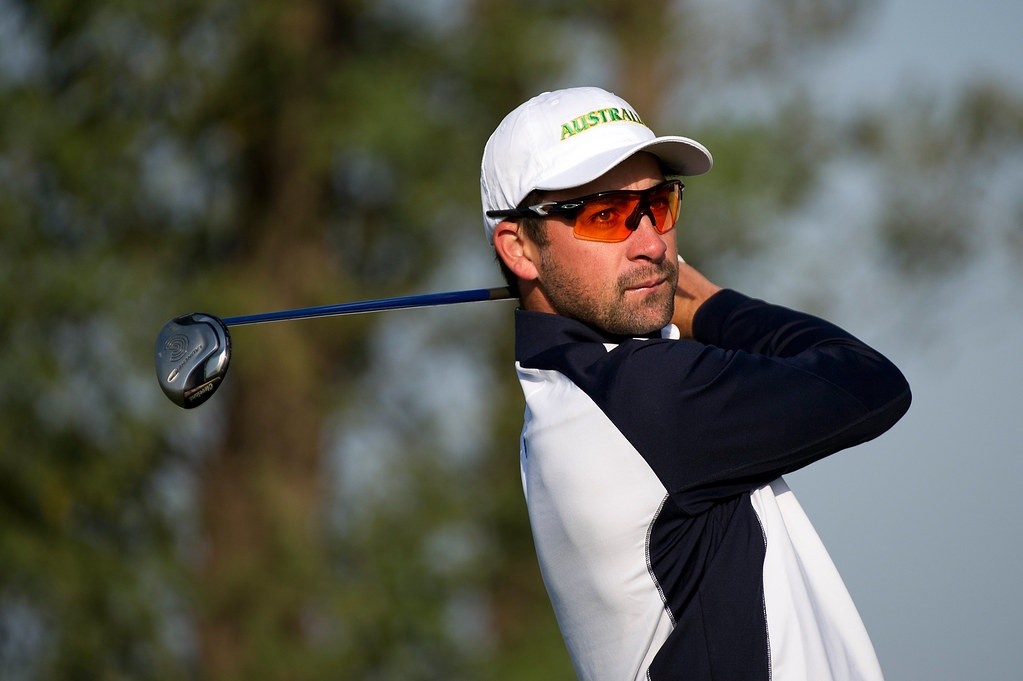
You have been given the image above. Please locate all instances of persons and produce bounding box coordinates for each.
[480,86,912,681]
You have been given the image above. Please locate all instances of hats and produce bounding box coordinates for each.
[480,89,715,245]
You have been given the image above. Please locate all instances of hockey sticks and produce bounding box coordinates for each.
[154,286,525,408]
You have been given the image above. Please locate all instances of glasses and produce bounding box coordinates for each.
[485,179,685,241]
